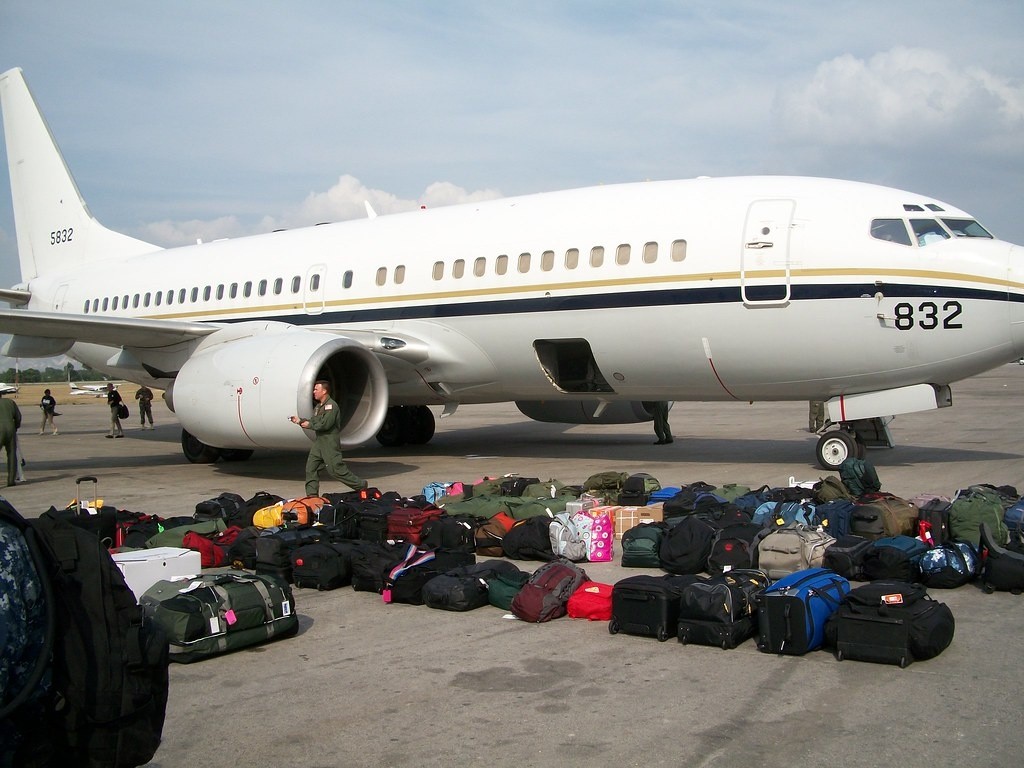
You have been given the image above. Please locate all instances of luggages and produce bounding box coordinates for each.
[58,476,118,550]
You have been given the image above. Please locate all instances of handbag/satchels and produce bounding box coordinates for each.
[119,405,129,419]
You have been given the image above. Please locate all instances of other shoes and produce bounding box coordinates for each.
[51,432,57,436]
[355,480,367,493]
[810,426,826,432]
[105,435,113,438]
[115,435,124,438]
[38,432,44,435]
[664,437,673,443]
[653,438,665,444]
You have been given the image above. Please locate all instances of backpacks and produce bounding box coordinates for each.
[0,500,171,768]
[113,456,1024,668]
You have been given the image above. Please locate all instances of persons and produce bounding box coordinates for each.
[38,389,58,435]
[287,381,368,499]
[135,385,154,430]
[0,391,21,487]
[105,383,124,438]
[650,401,674,445]
[809,401,826,433]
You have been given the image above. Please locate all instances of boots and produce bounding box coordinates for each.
[141,424,145,430]
[151,423,155,430]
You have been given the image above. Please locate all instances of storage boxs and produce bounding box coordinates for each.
[615,506,644,540]
[637,502,664,524]
[589,505,622,536]
[111,547,202,605]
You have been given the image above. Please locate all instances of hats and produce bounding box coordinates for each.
[0,383,14,391]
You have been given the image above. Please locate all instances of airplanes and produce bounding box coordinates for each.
[68,380,122,399]
[0,64,1023,471]
